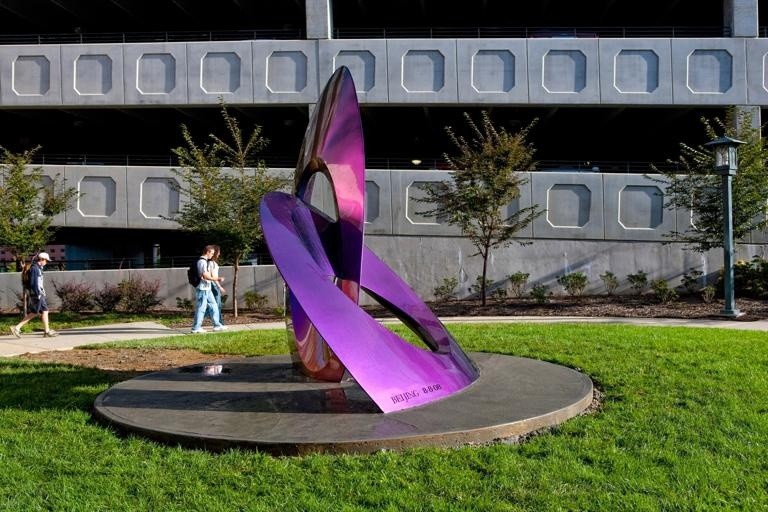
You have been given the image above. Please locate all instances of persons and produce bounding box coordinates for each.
[188,245,227,334]
[207,245,226,326]
[9,252,60,338]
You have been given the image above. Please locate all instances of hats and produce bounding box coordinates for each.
[38,252,53,262]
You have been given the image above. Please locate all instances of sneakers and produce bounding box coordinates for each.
[44,330,56,337]
[191,328,206,333]
[9,325,22,338]
[213,325,226,331]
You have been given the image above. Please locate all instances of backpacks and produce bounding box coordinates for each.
[187,258,208,287]
